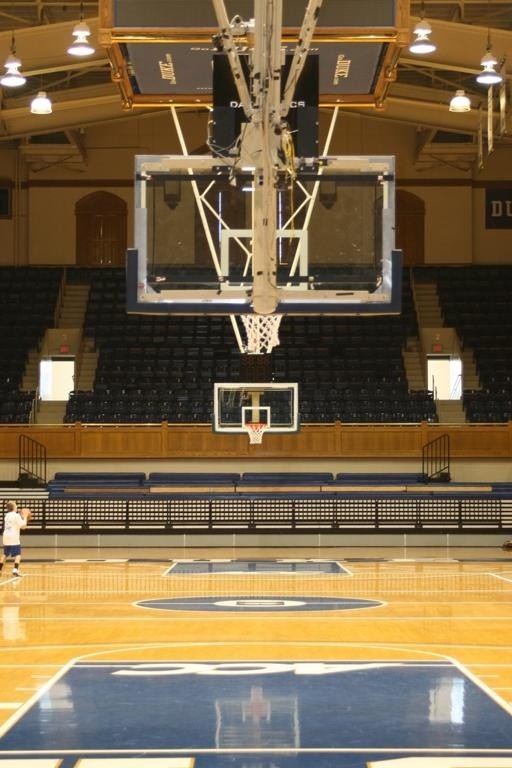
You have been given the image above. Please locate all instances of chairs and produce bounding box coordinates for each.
[1,265,511,425]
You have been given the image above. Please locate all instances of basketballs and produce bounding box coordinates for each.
[20,508,32,521]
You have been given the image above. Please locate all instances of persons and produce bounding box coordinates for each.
[0,501,31,576]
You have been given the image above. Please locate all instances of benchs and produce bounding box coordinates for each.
[47,472,512,499]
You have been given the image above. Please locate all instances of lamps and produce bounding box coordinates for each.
[408,0,503,114]
[0,1,94,117]
